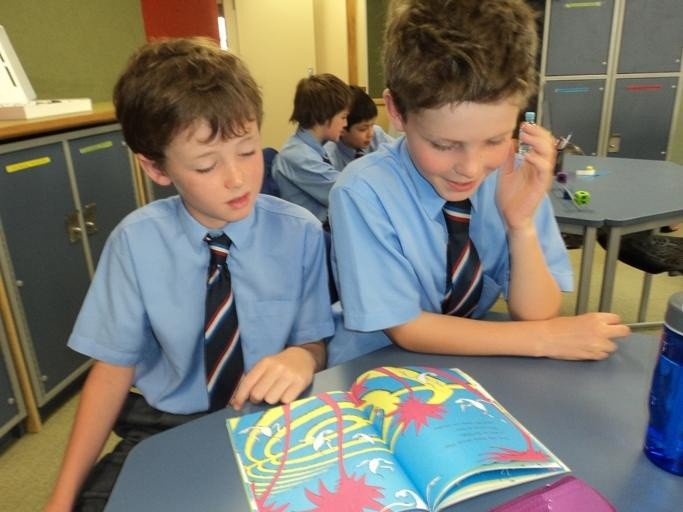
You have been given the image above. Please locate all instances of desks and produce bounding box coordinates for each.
[102,310,682,512]
[545,154,683,322]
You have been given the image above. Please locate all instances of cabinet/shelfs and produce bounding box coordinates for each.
[0,122,156,443]
[536,1,683,161]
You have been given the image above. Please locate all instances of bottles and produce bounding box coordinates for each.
[642,292,682,477]
[519,112,535,154]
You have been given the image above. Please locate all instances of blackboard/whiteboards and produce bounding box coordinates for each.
[365,0,389,104]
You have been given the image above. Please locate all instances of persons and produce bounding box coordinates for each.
[30,32,335,512]
[272,73,355,274]
[321,86,394,179]
[327,2,631,367]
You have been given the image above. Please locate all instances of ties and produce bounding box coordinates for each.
[442,198,483,318]
[355,148,364,158]
[202,234,244,416]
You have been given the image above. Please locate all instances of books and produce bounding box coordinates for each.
[222,367,572,510]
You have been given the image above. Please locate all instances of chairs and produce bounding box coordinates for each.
[596,232,683,322]
[258,146,282,198]
